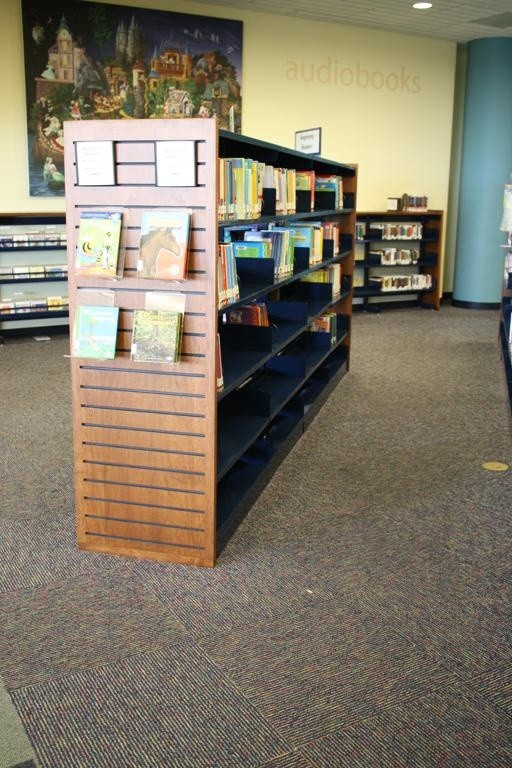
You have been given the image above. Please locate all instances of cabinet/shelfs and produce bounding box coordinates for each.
[355,208,444,311]
[497,184,512,411]
[0,212,67,346]
[63,116,358,572]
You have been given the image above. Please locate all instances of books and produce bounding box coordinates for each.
[217,335,224,392]
[219,157,342,346]
